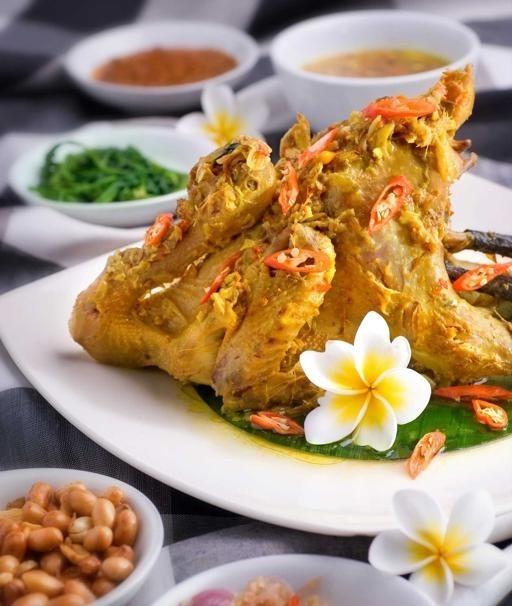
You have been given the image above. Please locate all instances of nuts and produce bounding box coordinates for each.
[1,480,137,606]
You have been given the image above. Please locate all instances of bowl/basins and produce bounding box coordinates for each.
[269,5,484,132]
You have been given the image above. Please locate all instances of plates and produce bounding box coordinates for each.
[63,17,261,114]
[1,170,512,545]
[7,124,230,230]
[0,465,166,606]
[149,552,438,606]
